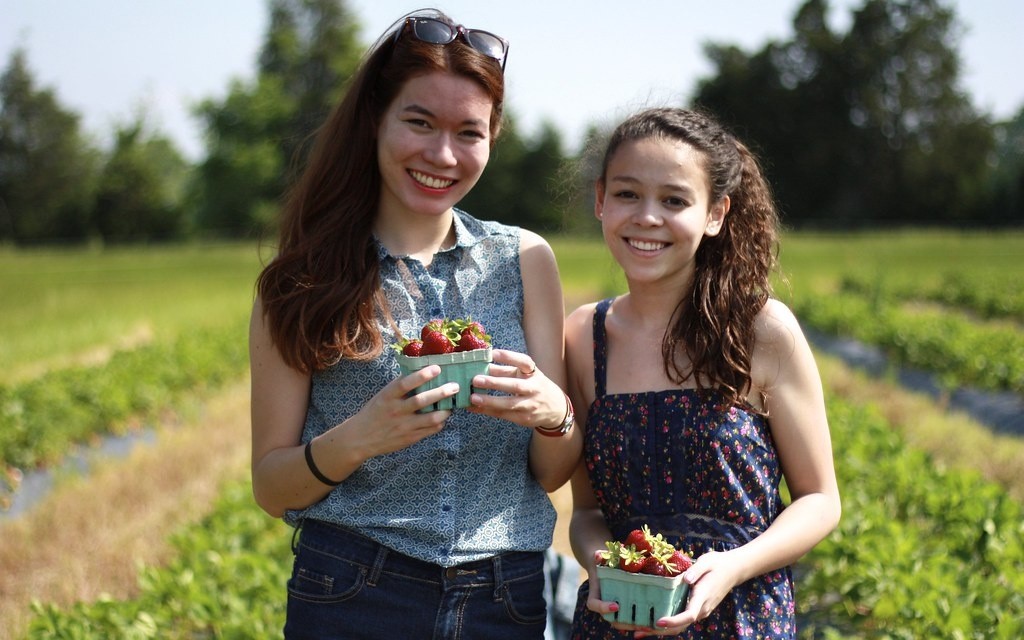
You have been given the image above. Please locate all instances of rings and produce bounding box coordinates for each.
[519,363,536,374]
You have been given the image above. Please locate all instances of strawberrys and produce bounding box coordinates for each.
[594,524,692,577]
[402,318,487,356]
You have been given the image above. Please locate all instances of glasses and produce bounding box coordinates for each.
[394,17,509,75]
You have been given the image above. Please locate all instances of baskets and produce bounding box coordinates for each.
[596,560,698,630]
[395,343,492,414]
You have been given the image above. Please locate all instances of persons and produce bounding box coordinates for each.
[250,6,586,640]
[562,108,842,640]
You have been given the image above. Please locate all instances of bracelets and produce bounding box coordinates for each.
[305,441,343,486]
[535,391,576,438]
